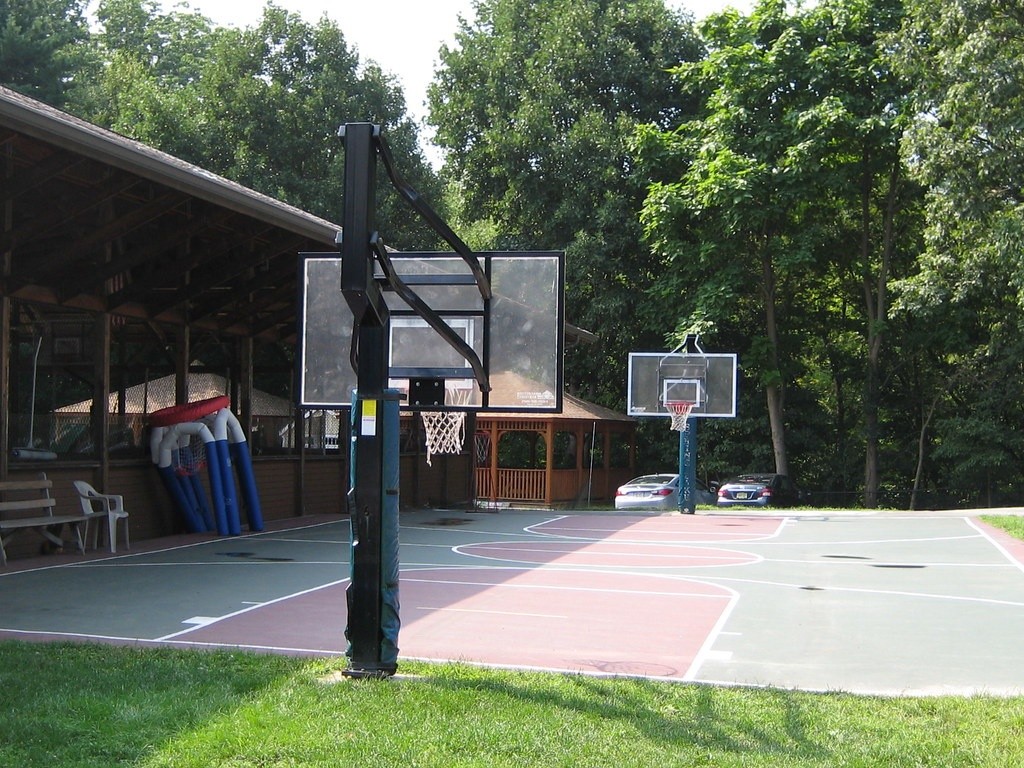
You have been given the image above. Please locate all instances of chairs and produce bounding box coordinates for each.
[73,481,130,553]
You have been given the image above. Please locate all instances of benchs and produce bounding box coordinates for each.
[0,472,89,569]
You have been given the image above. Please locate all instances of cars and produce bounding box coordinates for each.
[716,474,813,509]
[614,472,718,510]
